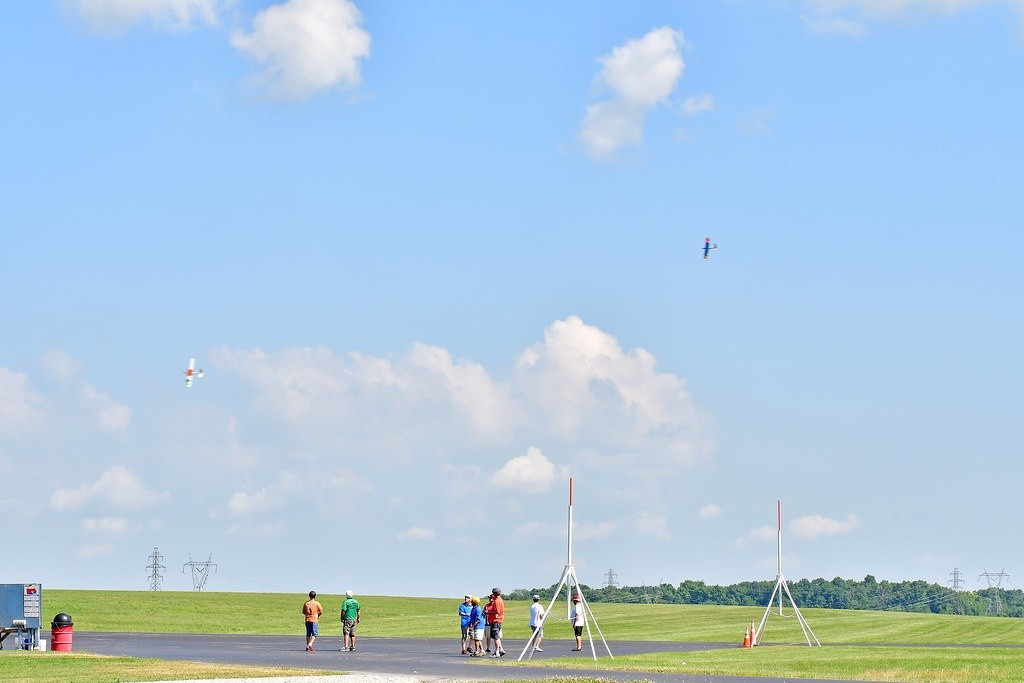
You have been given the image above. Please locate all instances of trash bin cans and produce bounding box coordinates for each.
[50,612,75,653]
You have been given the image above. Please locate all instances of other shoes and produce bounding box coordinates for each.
[534,646,543,652]
[486,648,491,652]
[467,647,474,653]
[490,654,500,658]
[306,645,314,651]
[462,650,467,654]
[350,646,356,651]
[339,646,350,652]
[500,650,506,656]
[470,651,479,657]
[572,649,581,651]
[478,650,486,657]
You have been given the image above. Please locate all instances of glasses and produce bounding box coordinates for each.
[488,596,490,598]
[465,595,470,599]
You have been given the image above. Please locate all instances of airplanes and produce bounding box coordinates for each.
[701,236,718,259]
[181,357,204,388]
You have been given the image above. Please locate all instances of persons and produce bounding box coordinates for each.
[458,587,505,658]
[340,590,361,652]
[529,595,545,652]
[302,591,323,653]
[570,595,585,651]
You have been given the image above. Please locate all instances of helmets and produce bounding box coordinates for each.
[572,595,581,601]
[309,591,316,599]
[346,590,353,598]
[492,587,501,595]
[490,594,495,598]
[465,593,472,600]
[471,597,480,604]
[533,595,540,600]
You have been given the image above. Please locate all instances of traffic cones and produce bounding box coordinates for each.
[740,627,750,647]
[749,621,760,646]
